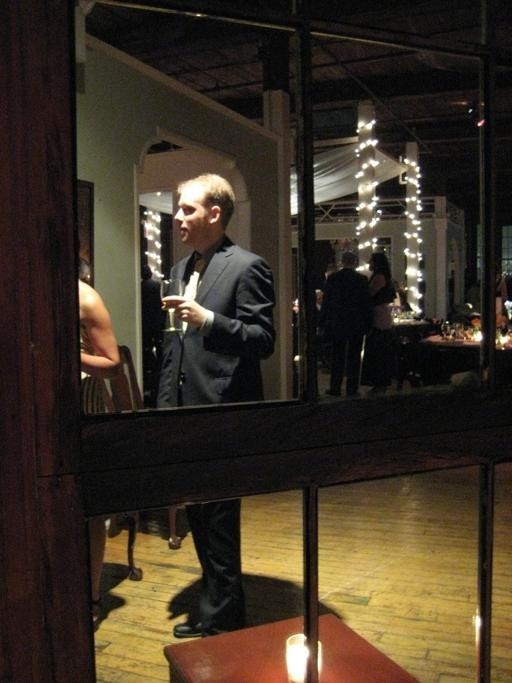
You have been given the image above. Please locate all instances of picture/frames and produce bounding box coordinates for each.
[77,180,94,290]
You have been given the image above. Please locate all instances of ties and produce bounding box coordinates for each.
[178,259,204,342]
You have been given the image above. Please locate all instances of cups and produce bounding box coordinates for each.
[286,633,322,683]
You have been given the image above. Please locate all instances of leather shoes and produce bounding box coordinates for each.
[174,619,203,637]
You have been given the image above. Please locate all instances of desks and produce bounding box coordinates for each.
[163,612,421,683]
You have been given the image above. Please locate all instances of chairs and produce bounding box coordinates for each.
[91,346,182,582]
[384,330,415,393]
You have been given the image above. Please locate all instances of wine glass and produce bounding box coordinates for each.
[160,279,185,332]
[441,322,451,342]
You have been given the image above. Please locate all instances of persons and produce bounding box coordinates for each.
[157,173,275,637]
[78,276,123,630]
[292,248,411,397]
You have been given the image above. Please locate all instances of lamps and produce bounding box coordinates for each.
[466,106,486,129]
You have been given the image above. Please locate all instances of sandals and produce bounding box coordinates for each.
[91,595,102,631]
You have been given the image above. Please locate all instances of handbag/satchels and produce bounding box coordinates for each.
[367,285,396,307]
